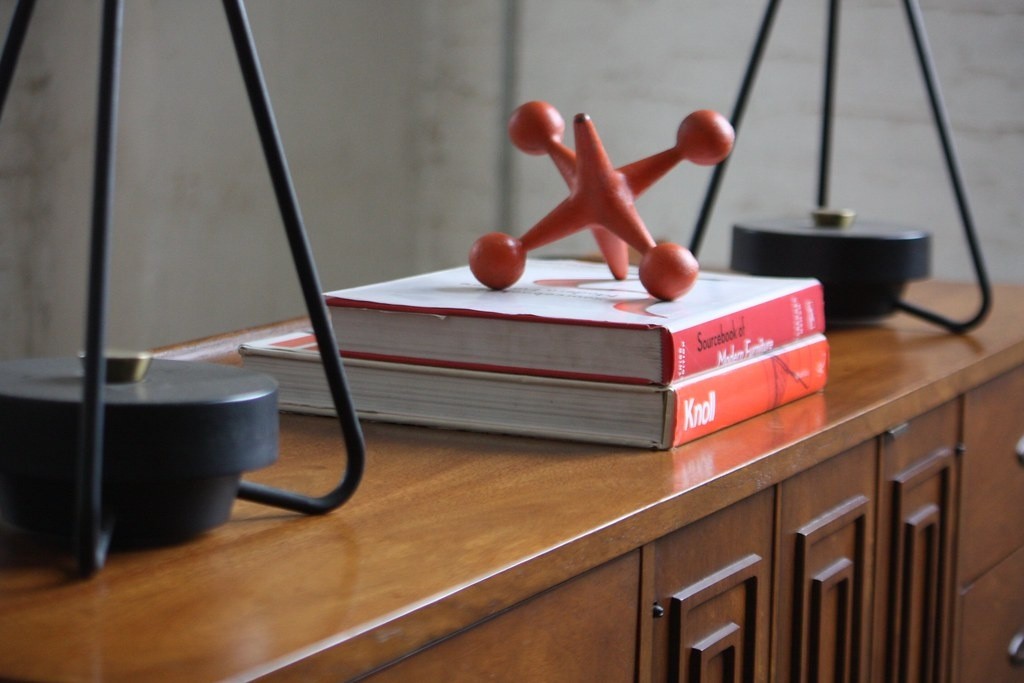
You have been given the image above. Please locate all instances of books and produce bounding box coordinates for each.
[323,258,826,389]
[237,330,831,451]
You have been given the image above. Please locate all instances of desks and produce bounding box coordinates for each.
[0,278,1024,683]
